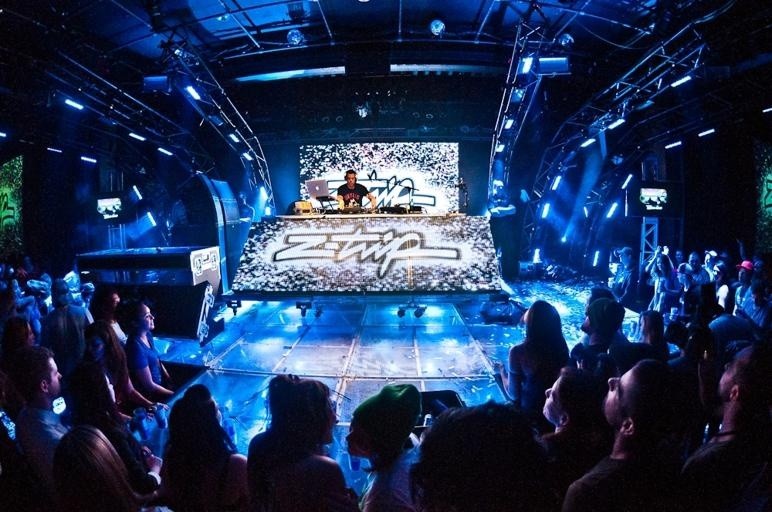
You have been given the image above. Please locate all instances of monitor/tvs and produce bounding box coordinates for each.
[88,191,136,225]
[627,181,684,218]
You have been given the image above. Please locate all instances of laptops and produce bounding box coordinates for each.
[305,180,338,201]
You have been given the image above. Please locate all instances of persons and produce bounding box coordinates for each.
[0,242,771,511]
[487,181,516,282]
[337,170,373,207]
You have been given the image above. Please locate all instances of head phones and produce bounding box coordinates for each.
[345,170,358,182]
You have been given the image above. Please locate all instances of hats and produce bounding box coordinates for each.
[588,298,625,333]
[704,250,717,258]
[81,283,94,294]
[617,247,632,256]
[353,383,422,440]
[676,263,693,278]
[735,260,753,271]
[52,280,73,305]
